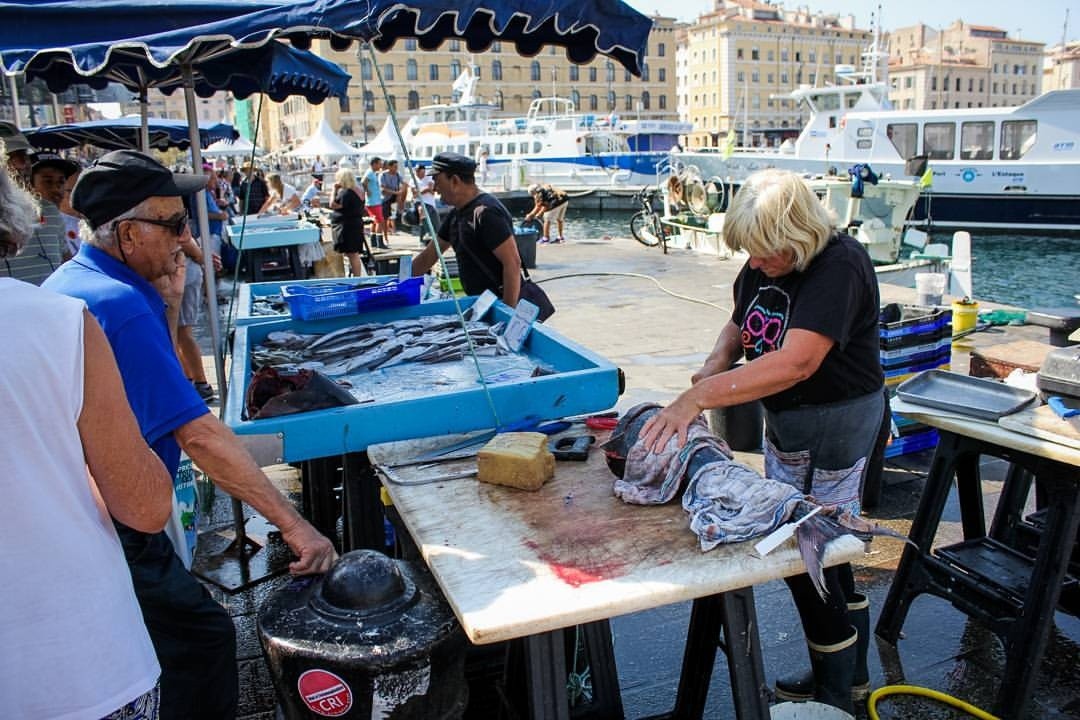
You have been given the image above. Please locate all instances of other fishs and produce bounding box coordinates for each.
[598,399,920,602]
[256,309,507,375]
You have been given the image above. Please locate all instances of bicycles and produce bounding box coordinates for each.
[628,183,671,256]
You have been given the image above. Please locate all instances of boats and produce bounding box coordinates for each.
[662,3,1080,237]
[408,93,694,212]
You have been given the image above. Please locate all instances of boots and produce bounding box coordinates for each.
[775,592,870,702]
[375,232,391,249]
[370,231,377,247]
[807,624,858,715]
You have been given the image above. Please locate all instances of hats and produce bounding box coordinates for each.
[30,151,79,179]
[425,151,477,184]
[0,120,28,153]
[69,149,211,230]
[240,161,257,173]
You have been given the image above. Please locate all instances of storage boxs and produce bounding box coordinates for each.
[282,275,423,320]
[437,256,462,293]
[878,303,952,456]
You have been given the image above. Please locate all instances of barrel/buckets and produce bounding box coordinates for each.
[951,301,978,337]
[769,700,855,720]
[514,231,537,268]
[915,272,945,306]
[708,363,763,452]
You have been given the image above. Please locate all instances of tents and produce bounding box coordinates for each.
[203,116,412,160]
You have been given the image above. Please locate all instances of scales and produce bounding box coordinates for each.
[969,309,1080,380]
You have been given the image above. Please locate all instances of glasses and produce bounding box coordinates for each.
[115,208,189,237]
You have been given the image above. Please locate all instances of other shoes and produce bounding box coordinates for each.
[538,236,550,242]
[550,237,565,244]
[193,383,216,403]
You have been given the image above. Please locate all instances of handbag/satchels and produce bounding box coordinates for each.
[520,278,555,323]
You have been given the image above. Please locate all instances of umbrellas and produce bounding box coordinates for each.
[0,38,355,581]
[20,117,239,175]
[4,0,655,107]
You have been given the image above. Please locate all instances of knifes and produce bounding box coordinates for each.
[560,411,619,423]
[1047,397,1080,432]
[415,413,574,471]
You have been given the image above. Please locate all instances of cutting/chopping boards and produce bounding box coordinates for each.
[998,396,1080,449]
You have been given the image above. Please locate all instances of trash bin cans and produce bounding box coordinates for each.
[878,300,954,460]
[512,227,539,269]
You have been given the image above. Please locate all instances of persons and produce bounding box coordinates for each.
[639,168,887,708]
[195,171,234,305]
[183,152,440,250]
[329,165,366,277]
[410,148,522,309]
[0,123,340,720]
[524,184,570,244]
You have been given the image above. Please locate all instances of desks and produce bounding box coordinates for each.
[243,243,304,283]
[874,396,1080,720]
[365,414,867,720]
